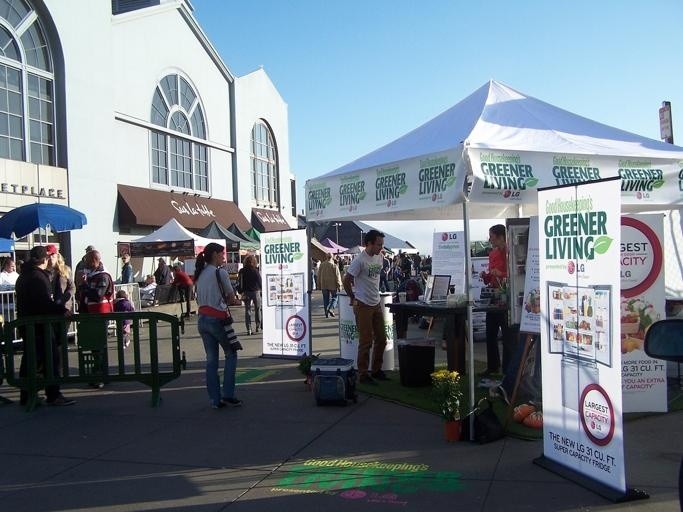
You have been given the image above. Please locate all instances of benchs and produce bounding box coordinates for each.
[140,284,172,307]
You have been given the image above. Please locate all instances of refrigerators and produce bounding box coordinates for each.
[546,278,615,415]
[267,270,306,330]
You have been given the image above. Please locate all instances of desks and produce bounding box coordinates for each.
[384,301,511,378]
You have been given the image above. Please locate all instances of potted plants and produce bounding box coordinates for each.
[298,351,320,392]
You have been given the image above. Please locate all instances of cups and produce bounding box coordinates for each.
[397,292,407,303]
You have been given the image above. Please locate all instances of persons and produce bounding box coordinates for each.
[1,243,434,348]
[73,249,115,390]
[478,222,512,379]
[341,228,389,384]
[191,240,243,410]
[14,245,78,413]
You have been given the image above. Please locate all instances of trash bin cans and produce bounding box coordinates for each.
[394,338,436,388]
[229,274,238,289]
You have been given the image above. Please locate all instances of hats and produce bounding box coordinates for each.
[46,245,56,254]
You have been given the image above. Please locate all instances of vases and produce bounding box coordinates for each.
[443,420,462,442]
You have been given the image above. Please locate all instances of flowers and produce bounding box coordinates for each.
[440,396,460,420]
[430,369,463,399]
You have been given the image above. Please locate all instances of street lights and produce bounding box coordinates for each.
[331,223,341,256]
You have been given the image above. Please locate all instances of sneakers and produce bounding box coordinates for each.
[46,397,76,406]
[329,310,335,317]
[255,328,261,332]
[210,401,226,408]
[248,330,252,335]
[222,397,242,407]
[91,383,108,388]
[326,314,329,318]
[20,397,45,406]
[372,369,387,379]
[359,374,373,384]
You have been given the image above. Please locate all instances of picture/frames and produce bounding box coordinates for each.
[430,274,452,301]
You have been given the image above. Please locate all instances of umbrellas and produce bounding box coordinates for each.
[0,202,89,247]
